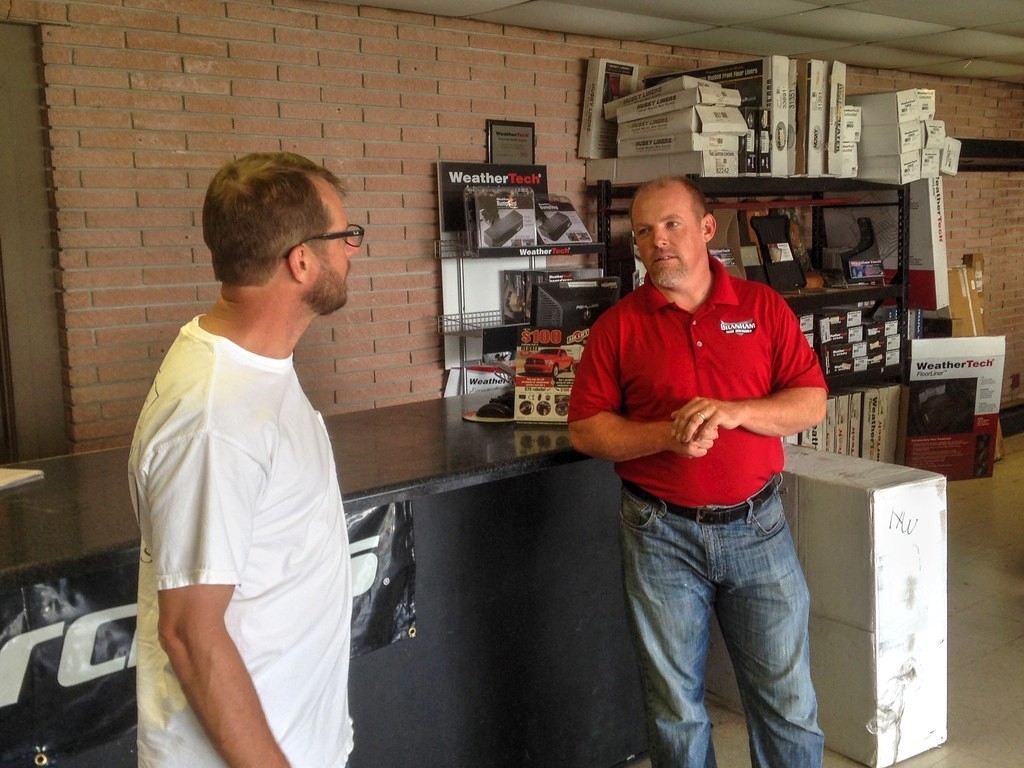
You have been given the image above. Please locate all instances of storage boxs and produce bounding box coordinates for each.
[464,54,1009,768]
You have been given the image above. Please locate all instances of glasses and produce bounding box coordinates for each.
[284,224,364,260]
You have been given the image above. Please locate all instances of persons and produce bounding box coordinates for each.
[568,176,830,768]
[126,154,367,768]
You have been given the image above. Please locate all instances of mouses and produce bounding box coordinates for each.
[476,403,514,418]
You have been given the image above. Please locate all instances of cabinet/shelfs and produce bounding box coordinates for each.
[431,160,606,372]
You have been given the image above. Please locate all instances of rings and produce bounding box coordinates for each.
[698,412,705,419]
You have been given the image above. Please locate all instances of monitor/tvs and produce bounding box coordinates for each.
[530,276,621,326]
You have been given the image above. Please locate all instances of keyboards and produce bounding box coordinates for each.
[490,389,515,410]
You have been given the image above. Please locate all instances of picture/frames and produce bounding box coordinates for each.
[486,119,536,165]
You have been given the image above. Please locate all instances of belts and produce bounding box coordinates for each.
[622,479,775,524]
